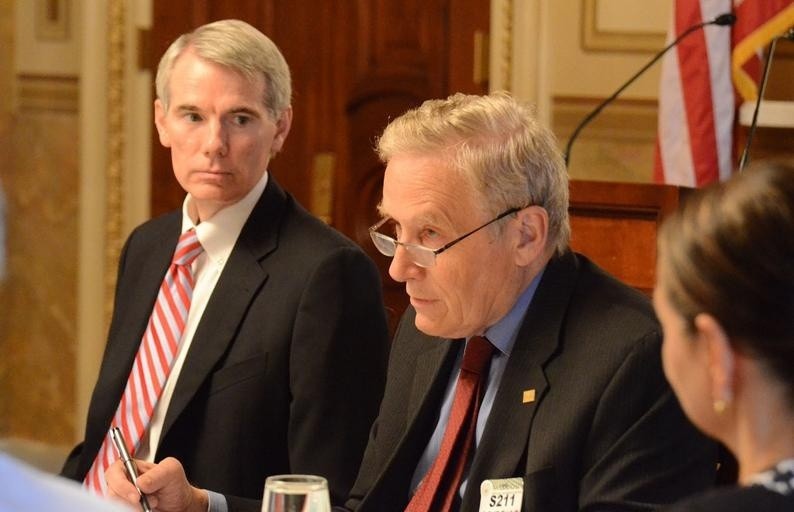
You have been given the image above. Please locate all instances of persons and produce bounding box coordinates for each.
[643,148,792,512]
[56,17,388,511]
[104,85,698,511]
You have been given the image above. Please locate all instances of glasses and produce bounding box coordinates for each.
[367,206,525,270]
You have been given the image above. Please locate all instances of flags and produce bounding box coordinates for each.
[649,0,793,191]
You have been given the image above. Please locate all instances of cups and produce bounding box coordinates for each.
[263,473,331,512]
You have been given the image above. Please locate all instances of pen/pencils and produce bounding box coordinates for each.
[110,427,150,512]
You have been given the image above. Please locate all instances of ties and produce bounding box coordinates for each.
[402,332,496,512]
[78,228,207,502]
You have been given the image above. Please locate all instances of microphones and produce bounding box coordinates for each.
[738,27,794,172]
[563,13,739,176]
